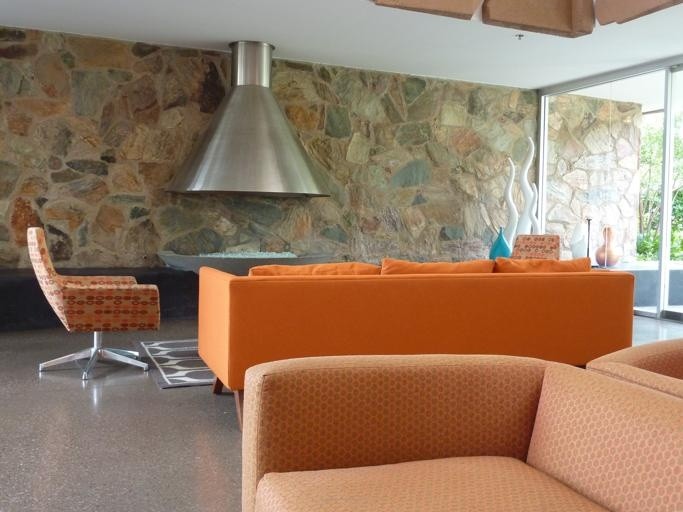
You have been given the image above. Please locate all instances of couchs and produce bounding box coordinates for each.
[194,267,637,432]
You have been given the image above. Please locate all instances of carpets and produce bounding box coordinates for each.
[130,335,219,392]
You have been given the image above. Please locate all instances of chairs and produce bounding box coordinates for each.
[238,354,680,511]
[584,338,681,399]
[507,229,561,261]
[23,227,162,383]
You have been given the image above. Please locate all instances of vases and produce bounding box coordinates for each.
[594,226,618,269]
[489,226,512,260]
